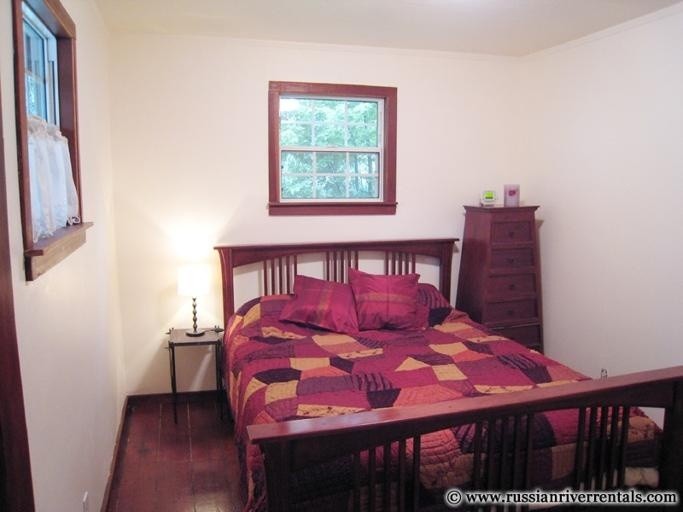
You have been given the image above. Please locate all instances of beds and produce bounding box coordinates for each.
[213,238,683,510]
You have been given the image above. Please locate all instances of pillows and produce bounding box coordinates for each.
[277,267,453,336]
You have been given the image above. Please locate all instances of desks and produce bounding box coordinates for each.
[166,328,225,423]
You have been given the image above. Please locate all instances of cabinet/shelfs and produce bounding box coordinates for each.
[456,204,544,355]
